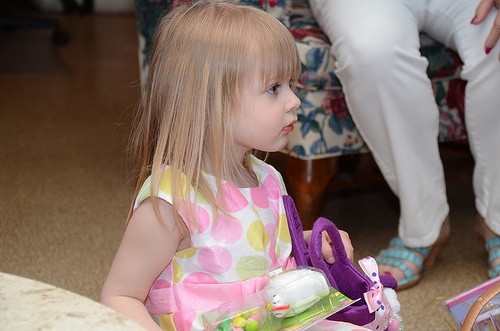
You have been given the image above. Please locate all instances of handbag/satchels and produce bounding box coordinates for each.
[282,195,401,331]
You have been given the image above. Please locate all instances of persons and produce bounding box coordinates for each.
[309,0,500,292]
[100,0,354,331]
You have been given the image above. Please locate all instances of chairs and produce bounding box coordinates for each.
[134,1,472,230]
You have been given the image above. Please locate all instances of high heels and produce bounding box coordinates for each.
[374,217,451,292]
[476,219,500,279]
